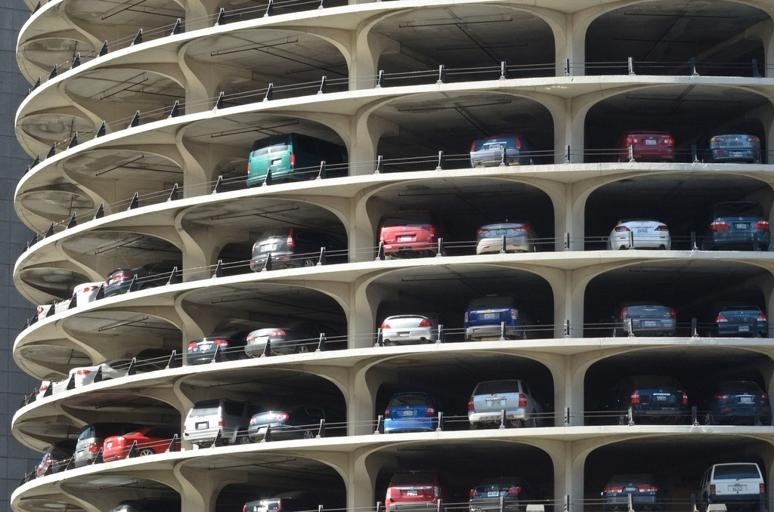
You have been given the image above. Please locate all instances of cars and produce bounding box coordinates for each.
[72,262,183,307]
[703,132,762,164]
[245,489,337,512]
[620,127,675,162]
[614,301,678,336]
[187,322,339,365]
[383,390,439,432]
[697,380,769,426]
[603,473,659,509]
[607,218,672,250]
[36,358,162,400]
[475,218,537,254]
[468,473,532,509]
[248,405,342,440]
[380,312,440,344]
[470,132,535,166]
[715,305,766,337]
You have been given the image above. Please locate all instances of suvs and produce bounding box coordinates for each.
[182,398,259,447]
[250,227,344,271]
[699,461,766,511]
[386,470,442,512]
[467,380,544,428]
[612,373,689,423]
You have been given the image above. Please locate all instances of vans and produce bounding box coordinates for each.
[380,209,461,259]
[464,294,536,339]
[690,201,770,251]
[36,421,178,476]
[245,133,346,186]
